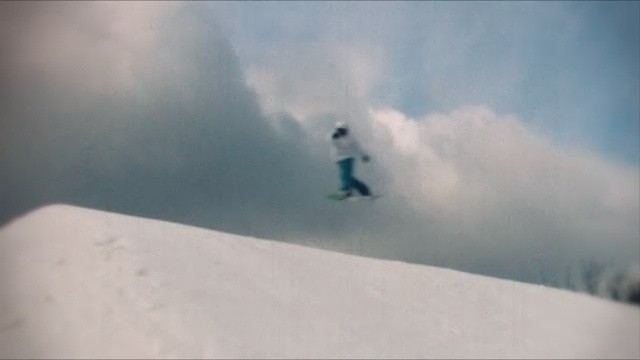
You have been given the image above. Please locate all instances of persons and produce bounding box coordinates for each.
[329,122,375,198]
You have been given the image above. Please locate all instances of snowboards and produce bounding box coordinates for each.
[327,193,378,202]
